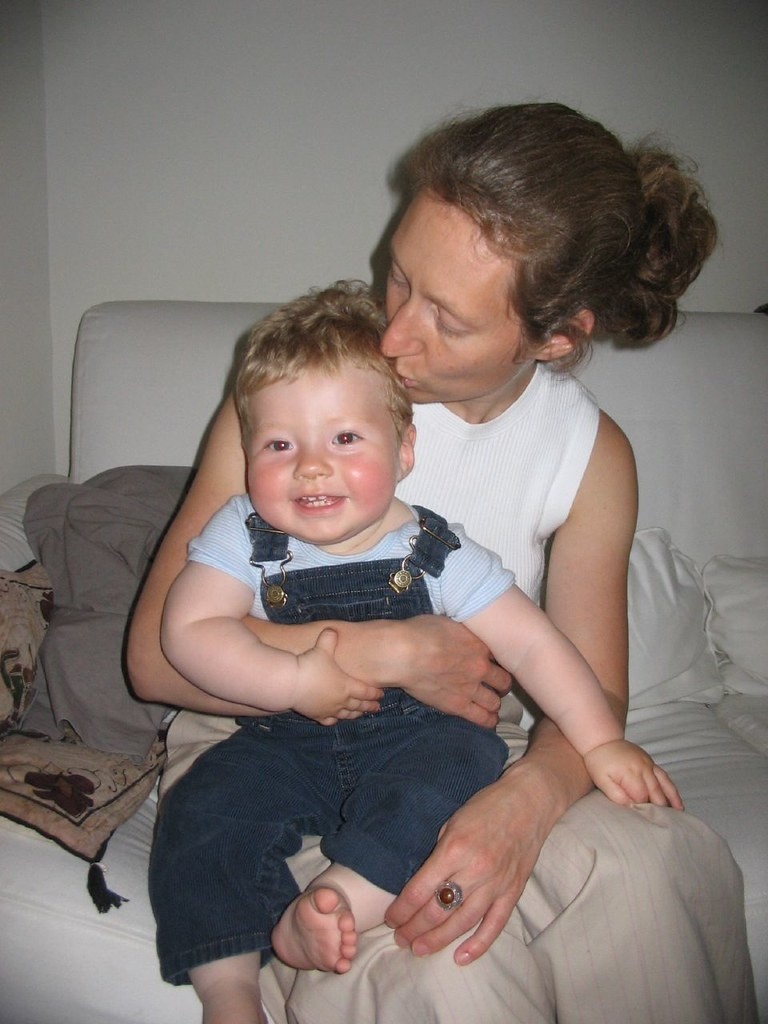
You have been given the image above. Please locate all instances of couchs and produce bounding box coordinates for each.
[0,296,768,1024]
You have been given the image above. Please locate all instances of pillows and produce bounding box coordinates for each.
[698,552,768,697]
[626,529,726,704]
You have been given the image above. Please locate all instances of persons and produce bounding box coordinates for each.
[148,289,685,1024]
[126,103,768,1024]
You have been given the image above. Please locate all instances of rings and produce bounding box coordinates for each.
[434,881,463,911]
